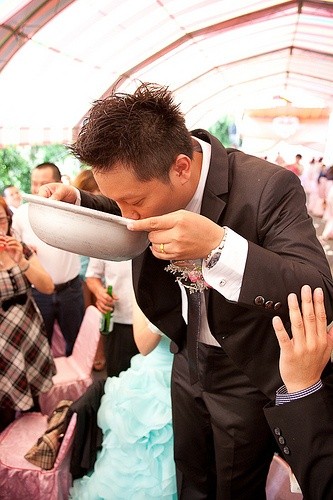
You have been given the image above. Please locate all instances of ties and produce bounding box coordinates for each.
[173,272,200,386]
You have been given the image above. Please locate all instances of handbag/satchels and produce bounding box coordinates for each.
[22,400,73,470]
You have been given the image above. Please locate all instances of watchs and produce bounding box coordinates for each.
[204,225,227,270]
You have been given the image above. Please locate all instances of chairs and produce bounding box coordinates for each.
[0,303,105,500]
[306,178,333,242]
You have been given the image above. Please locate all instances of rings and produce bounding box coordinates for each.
[160,243,166,254]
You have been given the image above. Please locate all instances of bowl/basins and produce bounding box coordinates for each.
[21,193,152,262]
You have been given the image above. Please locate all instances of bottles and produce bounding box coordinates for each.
[101,285,115,334]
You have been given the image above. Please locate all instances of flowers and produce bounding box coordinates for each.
[164,257,211,294]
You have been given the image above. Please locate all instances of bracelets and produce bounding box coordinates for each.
[21,263,30,272]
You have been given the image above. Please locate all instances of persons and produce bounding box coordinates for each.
[38,82,333,500]
[72,170,139,378]
[10,163,86,357]
[67,300,178,499]
[3,185,23,215]
[0,197,57,435]
[263,151,333,240]
[263,286,333,500]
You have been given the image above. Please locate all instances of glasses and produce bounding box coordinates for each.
[0,216,9,224]
